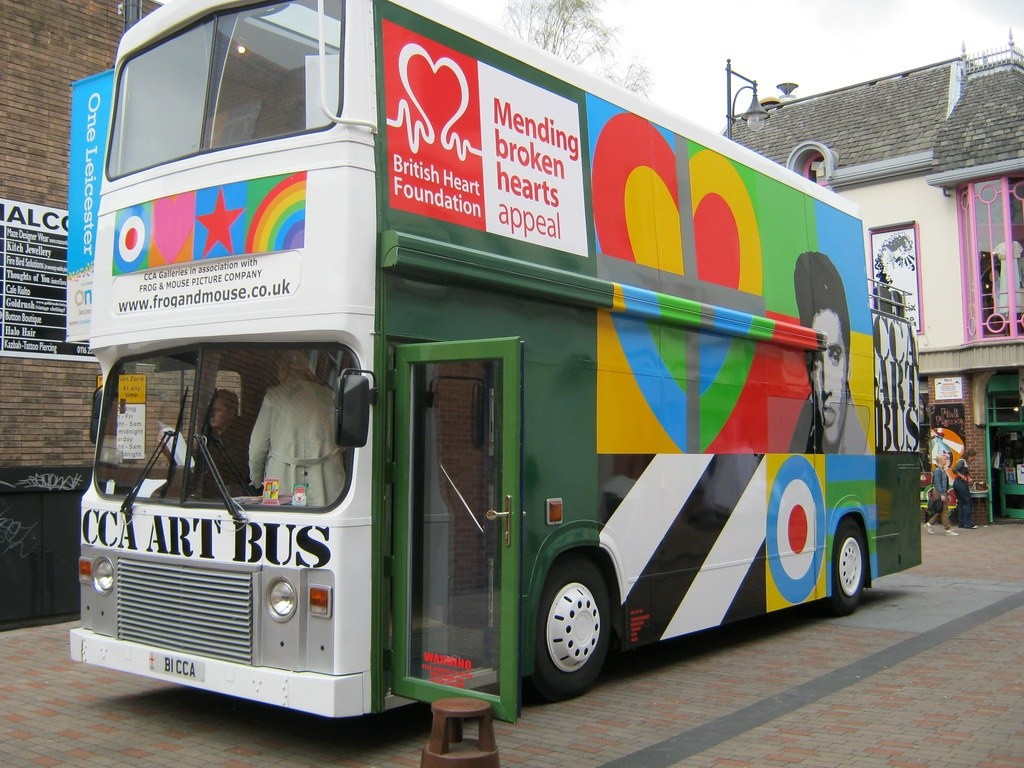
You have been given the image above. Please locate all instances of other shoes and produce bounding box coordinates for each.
[972,525,978,529]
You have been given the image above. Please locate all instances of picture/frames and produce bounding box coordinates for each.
[869,223,923,335]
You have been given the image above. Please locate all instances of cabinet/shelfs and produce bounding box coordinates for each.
[0,467,92,622]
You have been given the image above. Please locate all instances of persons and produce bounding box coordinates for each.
[158,390,263,499]
[922,454,959,536]
[250,352,346,507]
[952,448,978,529]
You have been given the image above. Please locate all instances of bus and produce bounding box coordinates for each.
[60,0,923,730]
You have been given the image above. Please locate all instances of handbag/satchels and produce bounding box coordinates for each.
[976,481,987,490]
[927,489,943,513]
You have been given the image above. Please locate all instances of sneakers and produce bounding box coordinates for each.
[944,529,959,535]
[923,522,935,535]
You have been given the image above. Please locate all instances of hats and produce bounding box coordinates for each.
[275,349,309,373]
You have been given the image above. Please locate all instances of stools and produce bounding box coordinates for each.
[420,697,500,768]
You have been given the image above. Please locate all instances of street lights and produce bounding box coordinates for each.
[721,55,802,145]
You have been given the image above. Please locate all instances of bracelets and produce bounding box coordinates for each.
[967,477,968,482]
[963,475,965,480]
